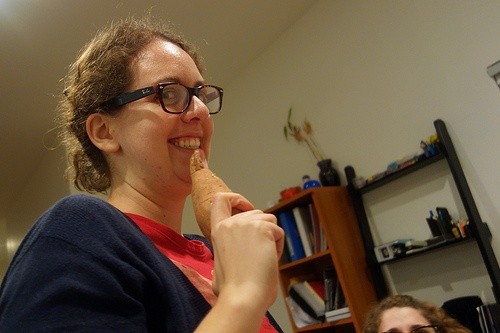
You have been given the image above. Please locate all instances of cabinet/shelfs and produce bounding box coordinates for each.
[264,188,378,333]
[344,120,500,308]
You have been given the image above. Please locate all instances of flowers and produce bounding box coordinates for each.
[283,104,324,161]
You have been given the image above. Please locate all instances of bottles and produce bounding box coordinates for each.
[302,175,321,190]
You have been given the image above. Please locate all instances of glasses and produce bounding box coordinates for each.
[103,82,224,114]
[383,325,439,333]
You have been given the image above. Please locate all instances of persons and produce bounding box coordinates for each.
[362,295,471,333]
[0,16,284,333]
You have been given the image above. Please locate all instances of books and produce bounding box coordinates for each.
[436,207,455,239]
[279,203,352,328]
[476,303,500,333]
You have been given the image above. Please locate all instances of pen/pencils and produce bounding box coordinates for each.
[429,209,444,225]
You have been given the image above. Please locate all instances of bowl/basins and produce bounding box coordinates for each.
[281,186,300,200]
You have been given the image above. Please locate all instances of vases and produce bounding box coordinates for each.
[317,158,340,186]
